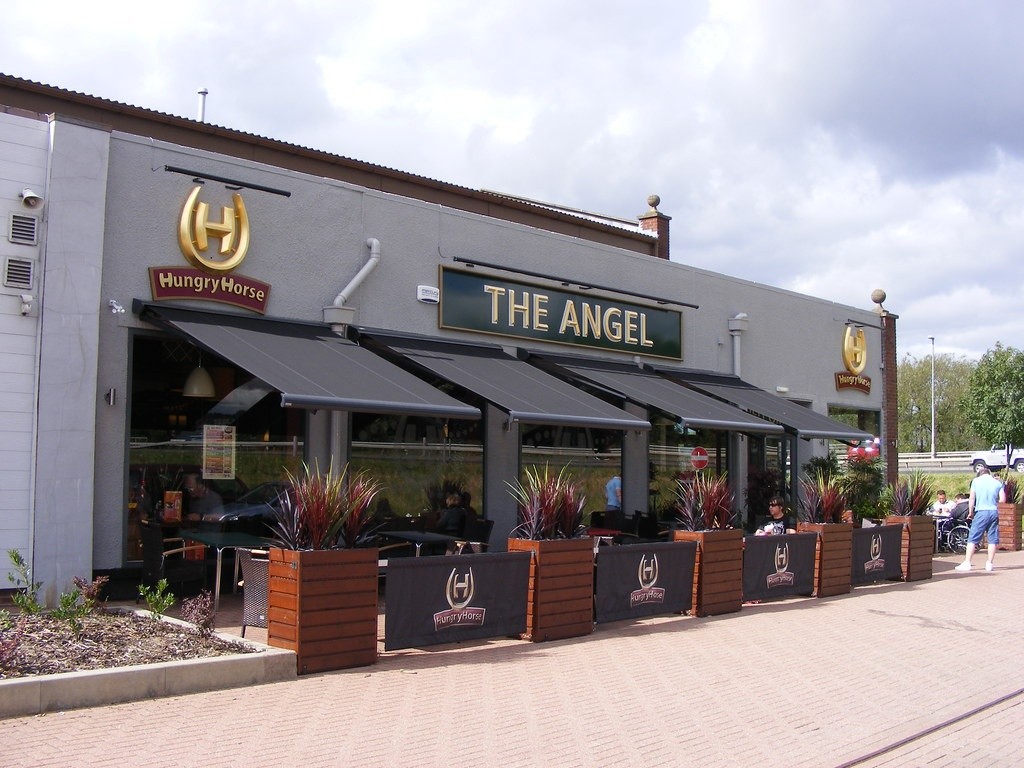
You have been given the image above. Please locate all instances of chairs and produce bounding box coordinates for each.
[636,509,670,539]
[237,546,270,639]
[619,509,641,543]
[136,518,208,599]
[589,509,618,530]
[361,540,412,578]
[453,517,496,554]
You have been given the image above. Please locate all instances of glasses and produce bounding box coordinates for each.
[768,503,781,507]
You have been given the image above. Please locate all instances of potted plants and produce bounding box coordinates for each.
[749,464,778,532]
[505,465,598,645]
[876,470,939,584]
[261,469,385,673]
[787,468,868,598]
[984,474,1023,552]
[669,467,751,616]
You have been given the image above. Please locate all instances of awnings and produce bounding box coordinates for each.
[146,305,481,422]
[531,352,785,439]
[362,331,651,432]
[656,369,874,448]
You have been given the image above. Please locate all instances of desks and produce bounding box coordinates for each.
[932,515,952,554]
[376,530,461,556]
[584,527,620,547]
[176,529,276,611]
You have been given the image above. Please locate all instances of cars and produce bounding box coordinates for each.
[129,464,297,561]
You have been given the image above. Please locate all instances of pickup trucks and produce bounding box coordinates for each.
[969,443,1024,473]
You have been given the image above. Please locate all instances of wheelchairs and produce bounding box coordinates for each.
[938,519,984,555]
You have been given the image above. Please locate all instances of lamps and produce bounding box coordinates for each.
[105,388,116,407]
[637,431,643,436]
[503,418,511,432]
[183,350,216,397]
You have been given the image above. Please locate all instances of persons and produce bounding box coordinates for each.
[955,469,1006,570]
[185,473,226,523]
[437,496,468,538]
[932,490,968,552]
[605,473,621,510]
[755,496,797,535]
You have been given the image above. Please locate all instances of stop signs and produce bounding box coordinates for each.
[691,446,709,470]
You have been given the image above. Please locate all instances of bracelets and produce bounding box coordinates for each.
[200,512,204,520]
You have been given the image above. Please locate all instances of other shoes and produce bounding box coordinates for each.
[985,561,993,571]
[955,561,971,570]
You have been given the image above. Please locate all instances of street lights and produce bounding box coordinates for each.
[928,337,936,461]
